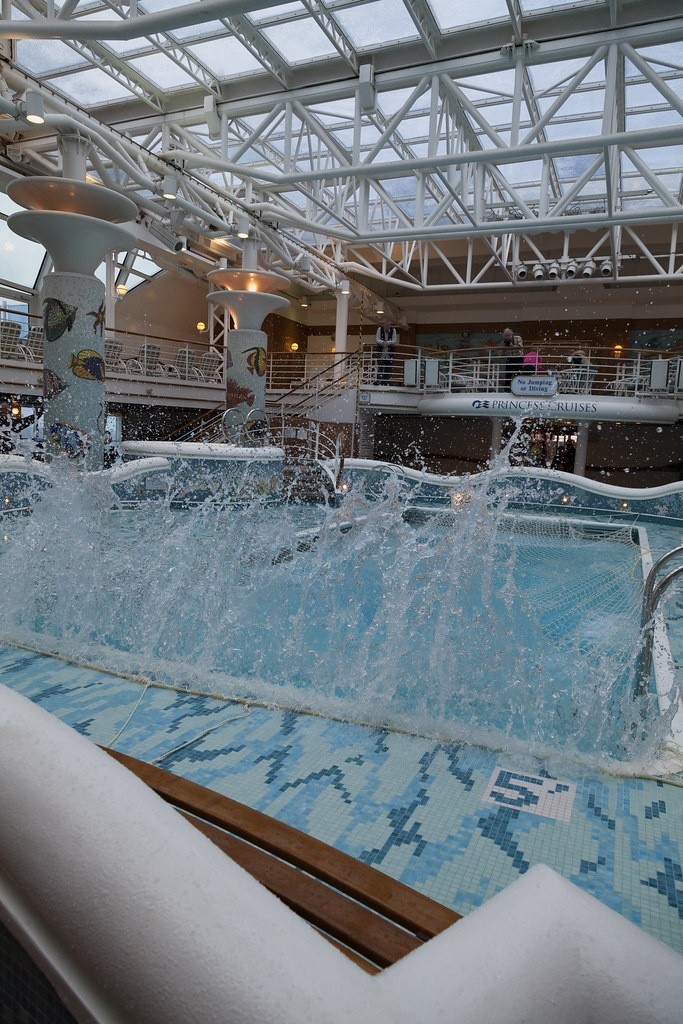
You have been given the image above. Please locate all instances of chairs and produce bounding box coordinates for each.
[165,349,202,380]
[0,321,29,362]
[193,351,221,382]
[22,325,45,362]
[104,337,128,374]
[554,368,599,394]
[127,344,168,378]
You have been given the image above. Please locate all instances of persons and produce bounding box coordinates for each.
[373,319,397,386]
[522,351,543,375]
[503,328,523,393]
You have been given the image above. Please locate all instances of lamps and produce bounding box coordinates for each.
[295,257,310,271]
[231,218,250,238]
[581,261,596,278]
[548,262,561,278]
[16,90,45,124]
[291,343,299,351]
[157,175,178,200]
[197,322,210,333]
[515,265,528,279]
[532,264,545,280]
[299,296,308,306]
[600,260,614,276]
[565,262,578,276]
[113,284,127,300]
[336,280,349,294]
[373,301,385,314]
